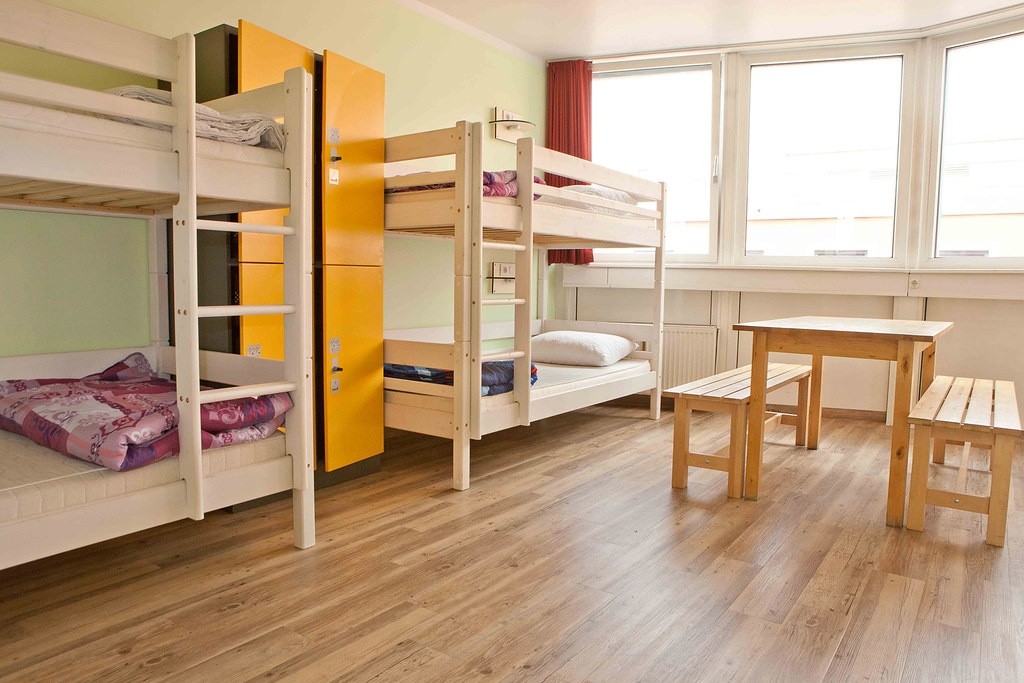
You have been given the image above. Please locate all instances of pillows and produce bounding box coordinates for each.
[531,330,639,366]
[539,184,639,215]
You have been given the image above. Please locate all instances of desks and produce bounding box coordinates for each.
[731,316,956,527]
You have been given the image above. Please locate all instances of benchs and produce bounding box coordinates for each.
[662,363,812,499]
[907,375,1024,547]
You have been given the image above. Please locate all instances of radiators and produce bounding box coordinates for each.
[644,325,717,391]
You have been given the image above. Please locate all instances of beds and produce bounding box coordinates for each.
[384,120,669,490]
[0,0,317,572]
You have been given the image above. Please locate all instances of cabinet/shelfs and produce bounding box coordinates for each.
[158,19,387,511]
